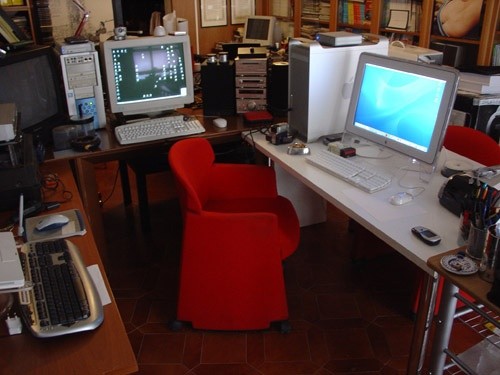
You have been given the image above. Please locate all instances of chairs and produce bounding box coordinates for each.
[167,135,301,336]
[443,125,500,168]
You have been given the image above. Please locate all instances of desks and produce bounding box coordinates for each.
[54,100,290,157]
[1,157,121,374]
[242,126,500,374]
[427,241,500,375]
[201,40,289,117]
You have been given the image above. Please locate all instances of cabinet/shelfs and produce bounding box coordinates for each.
[255,0,500,69]
[0,1,39,55]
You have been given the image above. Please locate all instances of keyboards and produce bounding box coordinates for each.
[305,150,392,194]
[18,238,104,338]
[114,115,206,145]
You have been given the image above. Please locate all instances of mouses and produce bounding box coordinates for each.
[33,213,70,232]
[389,192,414,205]
[211,117,228,128]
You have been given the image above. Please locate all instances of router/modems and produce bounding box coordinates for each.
[61,40,95,54]
[243,110,273,127]
[315,32,363,46]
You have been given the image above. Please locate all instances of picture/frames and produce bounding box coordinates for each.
[200,0,228,28]
[230,0,255,25]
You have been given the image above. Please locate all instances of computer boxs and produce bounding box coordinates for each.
[287,33,389,142]
[59,49,107,130]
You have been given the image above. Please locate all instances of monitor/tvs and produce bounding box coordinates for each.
[243,14,282,47]
[99,33,195,123]
[344,51,460,166]
[0,44,66,142]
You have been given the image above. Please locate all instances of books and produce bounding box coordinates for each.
[453,63,500,97]
[338,2,375,25]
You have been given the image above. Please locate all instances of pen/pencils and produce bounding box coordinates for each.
[470,182,500,230]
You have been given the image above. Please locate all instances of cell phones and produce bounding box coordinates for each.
[411,225,442,246]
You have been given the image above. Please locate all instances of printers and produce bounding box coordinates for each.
[437,164,500,219]
